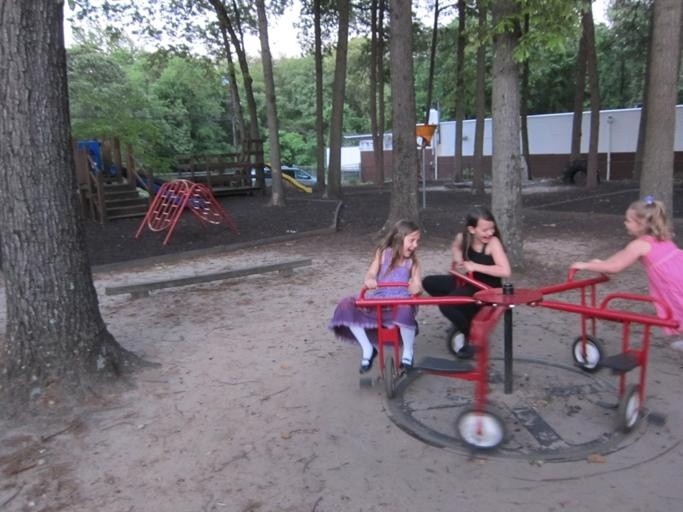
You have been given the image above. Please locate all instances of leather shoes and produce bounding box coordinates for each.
[400,356,414,369]
[359,346,377,373]
[445,324,476,360]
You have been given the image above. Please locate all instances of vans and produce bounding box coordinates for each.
[251,164,317,187]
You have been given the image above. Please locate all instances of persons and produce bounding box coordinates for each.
[420,203,511,360]
[327,218,424,374]
[568,194,681,352]
